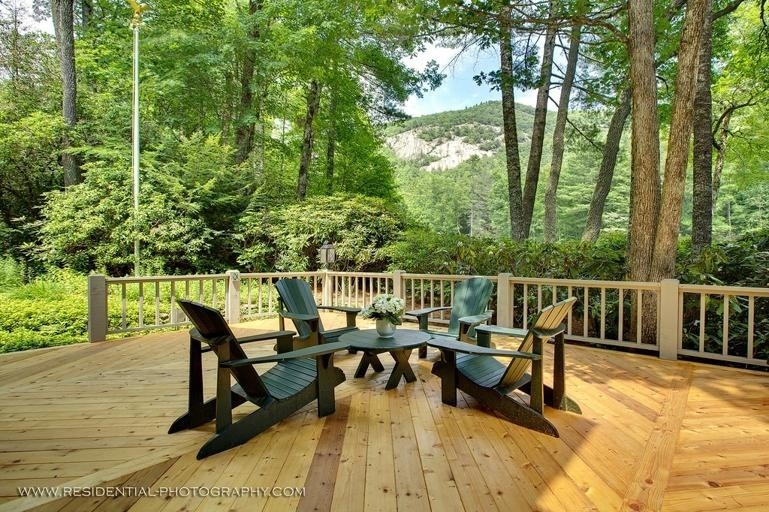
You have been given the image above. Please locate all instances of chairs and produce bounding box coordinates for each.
[167,276,580,461]
